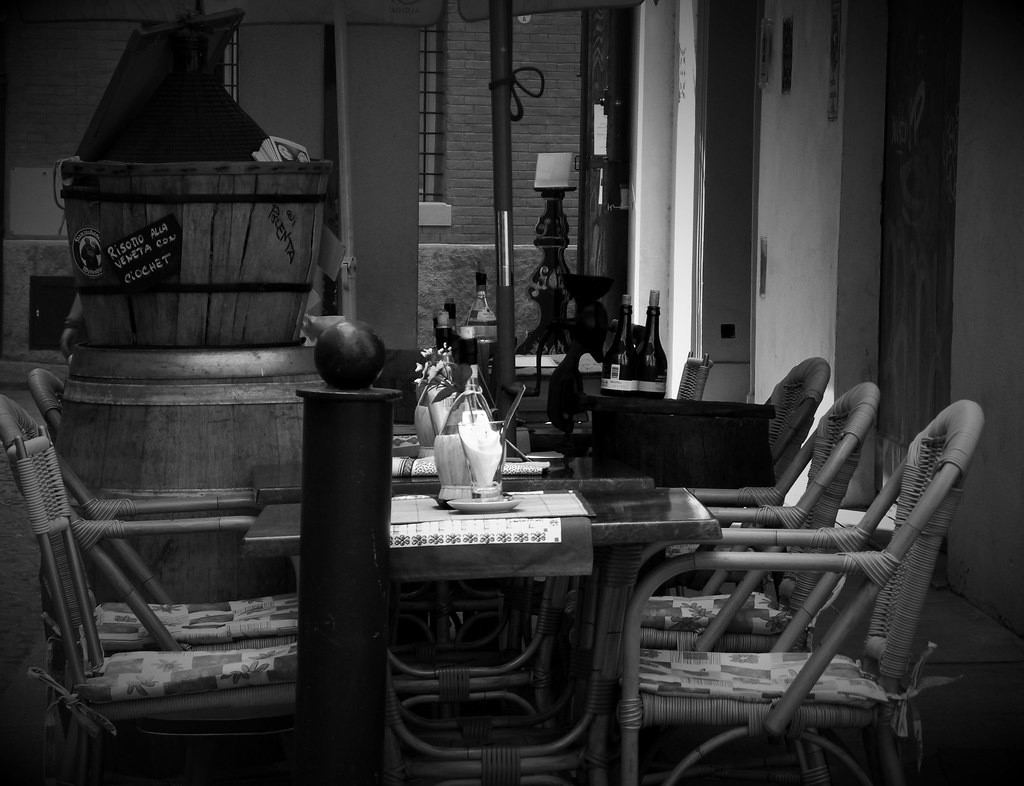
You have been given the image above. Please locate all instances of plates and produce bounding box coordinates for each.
[447,495,525,512]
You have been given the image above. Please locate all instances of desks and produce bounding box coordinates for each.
[247,485,723,786]
[247,451,598,716]
[483,350,601,426]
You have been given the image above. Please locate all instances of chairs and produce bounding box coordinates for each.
[2,354,987,785]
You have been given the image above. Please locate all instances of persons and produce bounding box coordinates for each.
[81,238,100,270]
[61,294,83,358]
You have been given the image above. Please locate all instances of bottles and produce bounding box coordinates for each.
[600,295,638,398]
[465,272,496,373]
[638,290,668,401]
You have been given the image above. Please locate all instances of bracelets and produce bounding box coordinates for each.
[64,317,80,329]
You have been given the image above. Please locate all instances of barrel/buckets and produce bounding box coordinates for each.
[59,160,336,344]
[53,346,323,605]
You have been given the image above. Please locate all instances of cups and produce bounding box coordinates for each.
[457,421,506,502]
